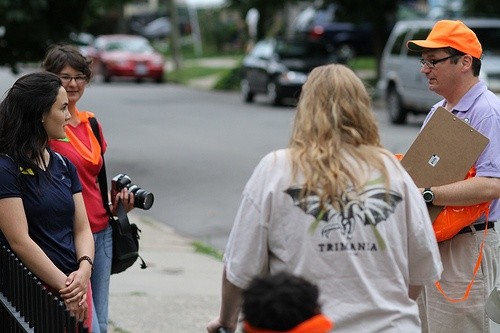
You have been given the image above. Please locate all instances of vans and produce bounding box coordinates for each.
[376,19,500,124]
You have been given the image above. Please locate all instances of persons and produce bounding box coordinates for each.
[242,272,335,333]
[406,20,500,333]
[43,46,135,333]
[207,65,442,333]
[0,73,95,333]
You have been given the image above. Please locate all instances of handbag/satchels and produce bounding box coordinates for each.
[110,218,141,275]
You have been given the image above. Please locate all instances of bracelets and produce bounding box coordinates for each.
[77,255,94,271]
[108,201,112,205]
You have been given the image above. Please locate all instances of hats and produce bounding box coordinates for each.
[406,19,483,59]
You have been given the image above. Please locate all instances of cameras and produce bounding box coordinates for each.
[111,174,154,210]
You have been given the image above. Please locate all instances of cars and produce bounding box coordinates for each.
[88,34,165,83]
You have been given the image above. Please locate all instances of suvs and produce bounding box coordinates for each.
[240,34,337,104]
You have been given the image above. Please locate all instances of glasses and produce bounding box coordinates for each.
[419,53,464,68]
[60,73,87,83]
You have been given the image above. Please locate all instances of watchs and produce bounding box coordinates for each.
[422,186,435,207]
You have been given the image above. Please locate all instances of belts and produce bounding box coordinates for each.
[457,221,495,233]
